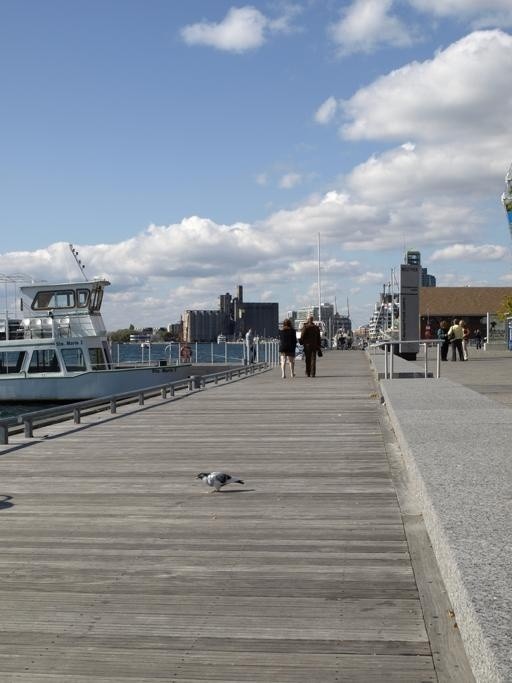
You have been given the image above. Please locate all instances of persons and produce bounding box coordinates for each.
[458,320,470,360]
[279,318,297,378]
[436,320,451,360]
[245,326,255,364]
[473,328,482,349]
[298,315,323,377]
[339,333,346,350]
[447,318,464,361]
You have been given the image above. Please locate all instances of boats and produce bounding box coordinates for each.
[0,243,192,401]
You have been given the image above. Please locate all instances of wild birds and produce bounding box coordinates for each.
[195,472,245,492]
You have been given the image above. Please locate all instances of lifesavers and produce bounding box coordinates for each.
[180,345,192,358]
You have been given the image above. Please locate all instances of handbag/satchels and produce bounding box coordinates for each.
[447,326,455,339]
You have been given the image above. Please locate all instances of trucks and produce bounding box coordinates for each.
[297,321,329,349]
